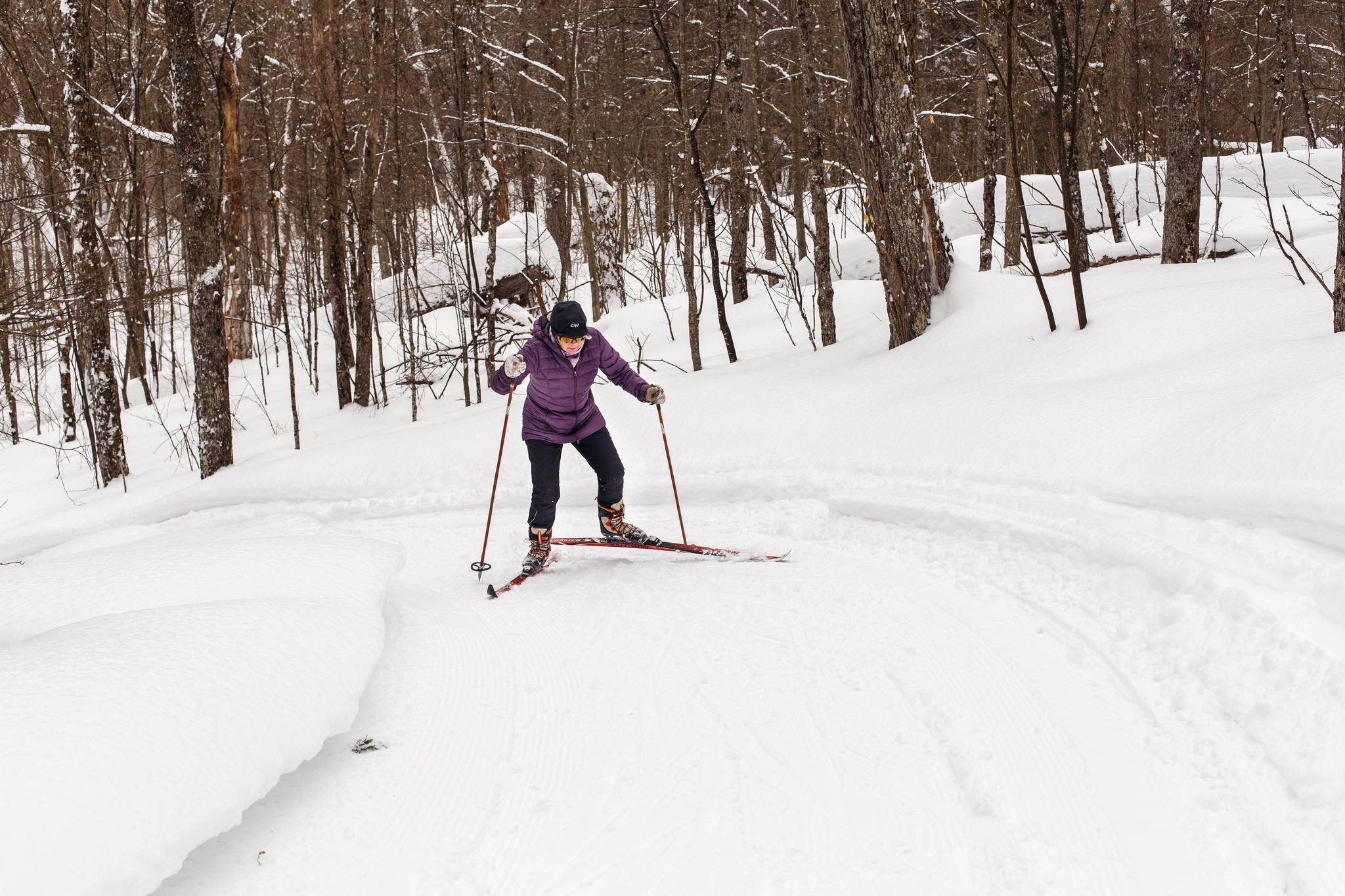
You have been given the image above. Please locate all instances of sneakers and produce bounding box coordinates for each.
[594,496,648,542]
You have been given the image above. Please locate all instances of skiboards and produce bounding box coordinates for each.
[487,537,792,597]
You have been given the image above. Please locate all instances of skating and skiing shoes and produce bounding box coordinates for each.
[522,526,551,571]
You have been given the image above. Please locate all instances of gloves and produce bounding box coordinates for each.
[645,384,666,405]
[504,354,527,378]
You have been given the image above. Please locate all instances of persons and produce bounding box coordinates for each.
[490,301,667,572]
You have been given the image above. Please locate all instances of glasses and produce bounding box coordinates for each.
[557,333,586,344]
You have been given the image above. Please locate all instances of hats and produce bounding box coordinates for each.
[550,300,588,338]
